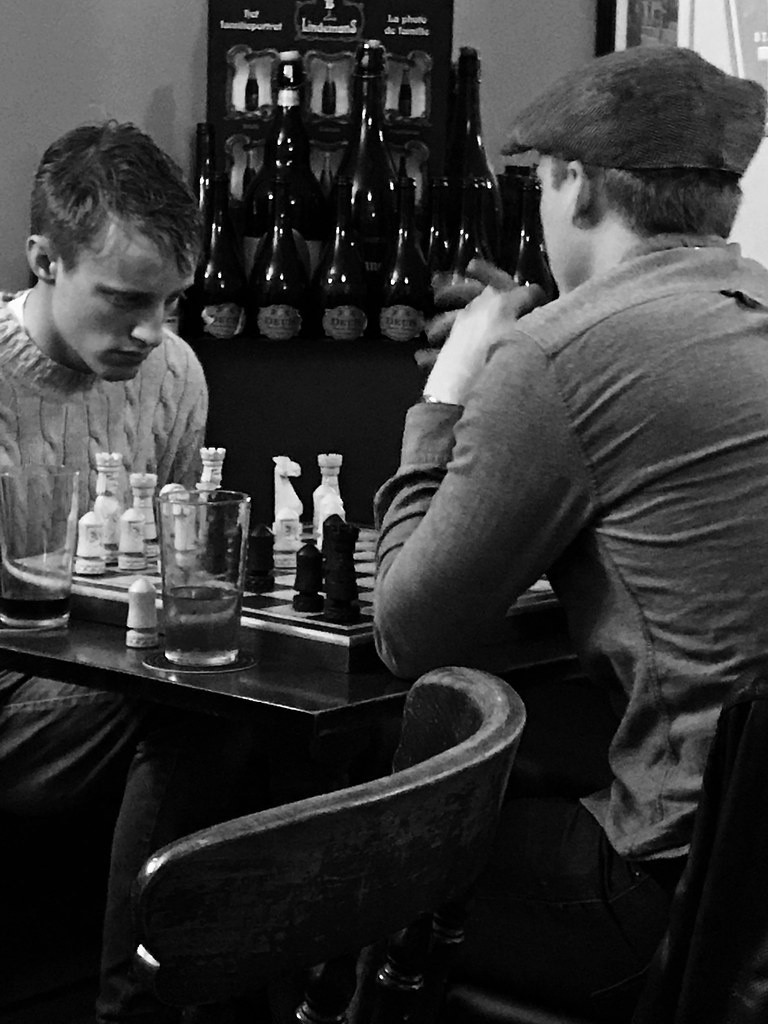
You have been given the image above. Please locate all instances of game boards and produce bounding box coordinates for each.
[14,516,567,683]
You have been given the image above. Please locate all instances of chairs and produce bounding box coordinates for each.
[100,666,530,1024]
[444,655,768,1023]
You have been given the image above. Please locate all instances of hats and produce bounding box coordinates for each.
[500,43,767,175]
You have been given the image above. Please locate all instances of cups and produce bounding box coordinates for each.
[153,489,251,667]
[0,463,78,630]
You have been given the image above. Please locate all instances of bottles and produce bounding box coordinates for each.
[177,38,556,348]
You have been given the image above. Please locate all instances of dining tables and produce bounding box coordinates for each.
[2,605,626,755]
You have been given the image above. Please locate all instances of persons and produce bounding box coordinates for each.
[368,44,768,1024]
[1,117,317,1024]
